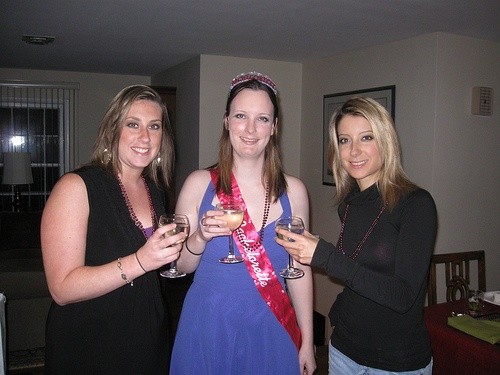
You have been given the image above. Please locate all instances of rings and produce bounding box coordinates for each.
[201,217,208,227]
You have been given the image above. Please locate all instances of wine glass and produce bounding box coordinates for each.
[158,213,191,280]
[215,201,246,263]
[275,216,305,279]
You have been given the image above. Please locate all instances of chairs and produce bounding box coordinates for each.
[428,250,486,308]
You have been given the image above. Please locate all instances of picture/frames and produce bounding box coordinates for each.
[322,84,396,187]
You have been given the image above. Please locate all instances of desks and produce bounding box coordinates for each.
[422,288,500,375]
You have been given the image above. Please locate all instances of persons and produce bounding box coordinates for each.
[274,96,439,375]
[40,85,189,375]
[169,72,318,375]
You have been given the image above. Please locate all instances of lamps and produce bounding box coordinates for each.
[1,152,34,214]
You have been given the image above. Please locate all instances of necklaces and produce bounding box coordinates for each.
[338,202,386,261]
[114,169,158,243]
[228,175,272,251]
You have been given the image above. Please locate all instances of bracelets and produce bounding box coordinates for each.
[117,258,133,286]
[185,238,204,255]
[135,252,148,273]
